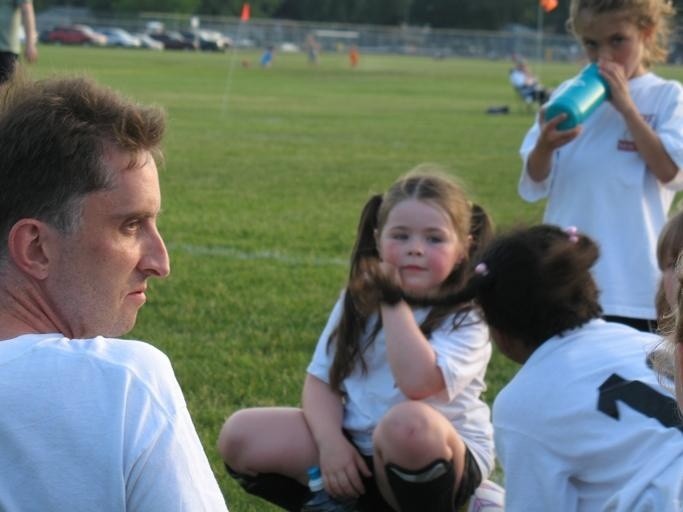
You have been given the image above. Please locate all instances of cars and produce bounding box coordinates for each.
[47,23,231,53]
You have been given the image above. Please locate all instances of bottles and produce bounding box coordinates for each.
[302,466,344,512]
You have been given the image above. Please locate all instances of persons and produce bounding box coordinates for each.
[0,70,227,512]
[512,60,552,104]
[215,170,498,510]
[513,1,682,334]
[656,214,683,405]
[0,1,39,81]
[363,222,683,510]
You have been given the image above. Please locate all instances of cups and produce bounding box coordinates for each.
[542,64,617,131]
